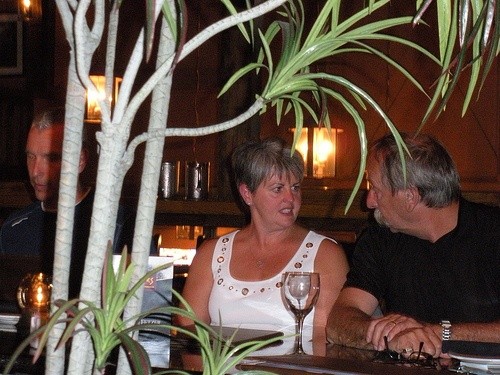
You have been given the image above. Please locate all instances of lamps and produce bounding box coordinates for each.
[287,108,347,183]
[84,65,124,128]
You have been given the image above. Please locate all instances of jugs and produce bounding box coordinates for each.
[159,160,176,199]
[185,161,208,201]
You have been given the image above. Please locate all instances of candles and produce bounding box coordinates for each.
[17,272,53,315]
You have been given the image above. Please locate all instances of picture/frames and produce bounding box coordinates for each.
[0,13,26,76]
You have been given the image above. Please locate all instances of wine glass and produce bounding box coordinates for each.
[282,272,320,354]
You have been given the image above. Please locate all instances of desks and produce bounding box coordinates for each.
[0,310,476,375]
[154,189,500,249]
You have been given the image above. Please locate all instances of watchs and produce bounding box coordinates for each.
[439,320,452,341]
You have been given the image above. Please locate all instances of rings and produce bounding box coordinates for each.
[403,347,412,353]
[390,320,396,326]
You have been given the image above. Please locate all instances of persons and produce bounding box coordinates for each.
[0,107,156,313]
[178,328,325,375]
[176,137,347,328]
[326,133,500,358]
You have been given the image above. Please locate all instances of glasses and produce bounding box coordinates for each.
[372,336,438,371]
